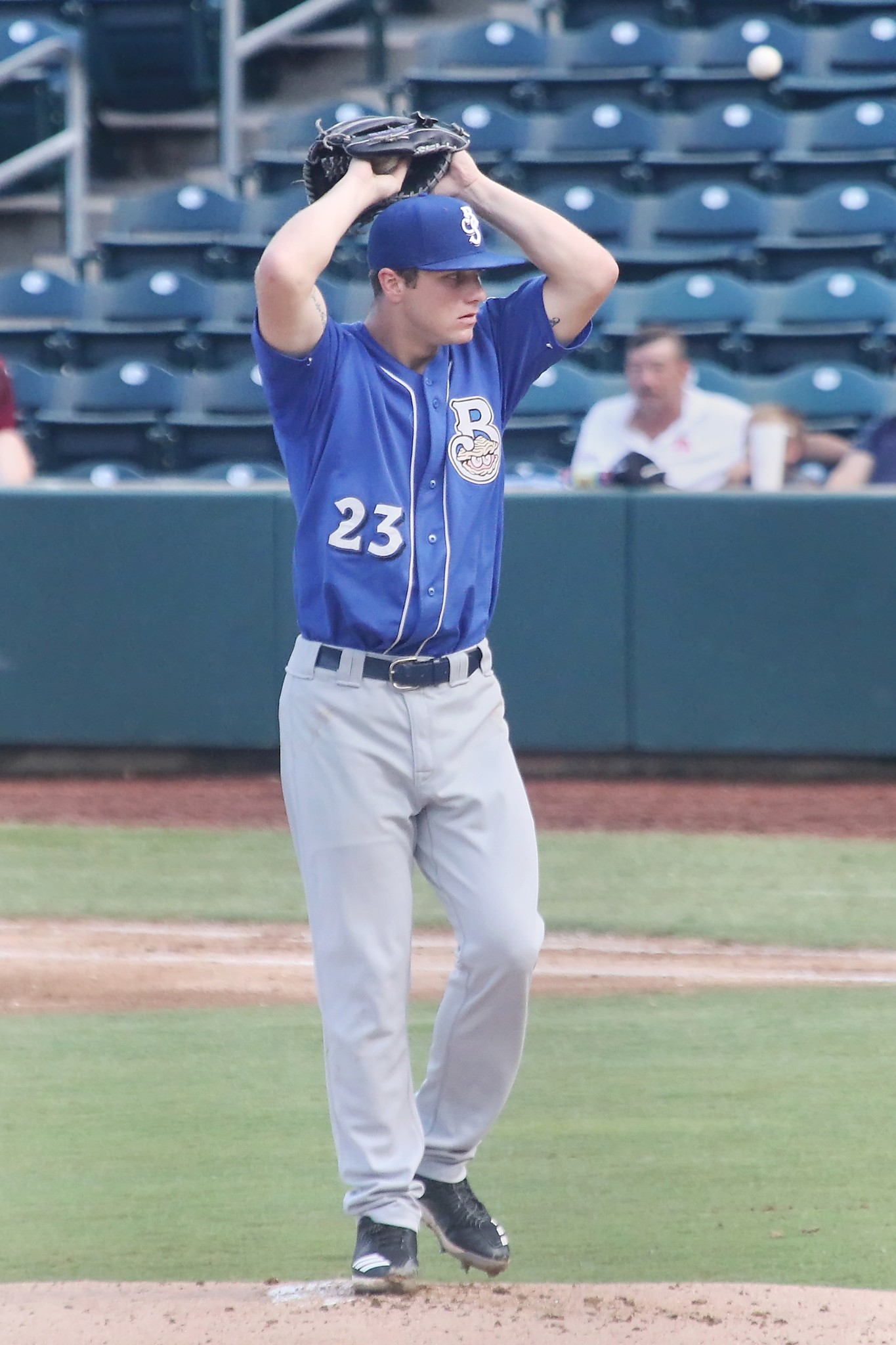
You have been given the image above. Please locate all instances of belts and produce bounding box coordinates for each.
[316,646,482,690]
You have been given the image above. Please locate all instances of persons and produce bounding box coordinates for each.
[719,406,820,489]
[252,154,619,1294]
[829,412,896,491]
[565,319,846,499]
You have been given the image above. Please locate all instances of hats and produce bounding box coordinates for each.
[368,193,524,273]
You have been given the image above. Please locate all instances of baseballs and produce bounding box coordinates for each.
[368,148,402,175]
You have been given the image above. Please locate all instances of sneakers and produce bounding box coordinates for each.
[412,1172,512,1279]
[350,1213,417,1294]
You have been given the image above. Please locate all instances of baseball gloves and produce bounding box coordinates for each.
[299,110,470,232]
